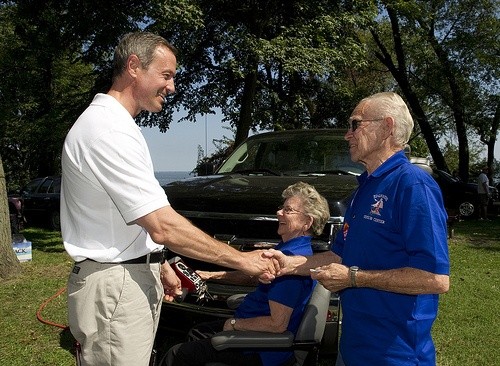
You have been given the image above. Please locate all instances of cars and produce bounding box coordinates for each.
[19,176,61,230]
[488,182,500,219]
[431,170,498,220]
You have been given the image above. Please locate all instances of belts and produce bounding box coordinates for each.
[86,252,163,264]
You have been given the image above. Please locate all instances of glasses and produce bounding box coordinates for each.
[278,205,307,216]
[348,119,385,132]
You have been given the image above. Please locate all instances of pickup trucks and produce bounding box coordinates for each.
[162,127,430,365]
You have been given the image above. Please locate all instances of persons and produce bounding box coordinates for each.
[60,30,280,365]
[257,92,450,366]
[159,181,330,366]
[477,166,492,222]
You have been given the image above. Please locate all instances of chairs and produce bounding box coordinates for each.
[205,282,331,366]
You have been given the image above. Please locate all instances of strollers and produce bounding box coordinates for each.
[8,193,37,239]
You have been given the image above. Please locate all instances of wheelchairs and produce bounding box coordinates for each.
[145,243,332,365]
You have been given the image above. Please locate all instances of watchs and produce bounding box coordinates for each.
[349,265,359,287]
[231,318,240,331]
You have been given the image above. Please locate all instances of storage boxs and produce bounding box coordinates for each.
[12,241,32,262]
